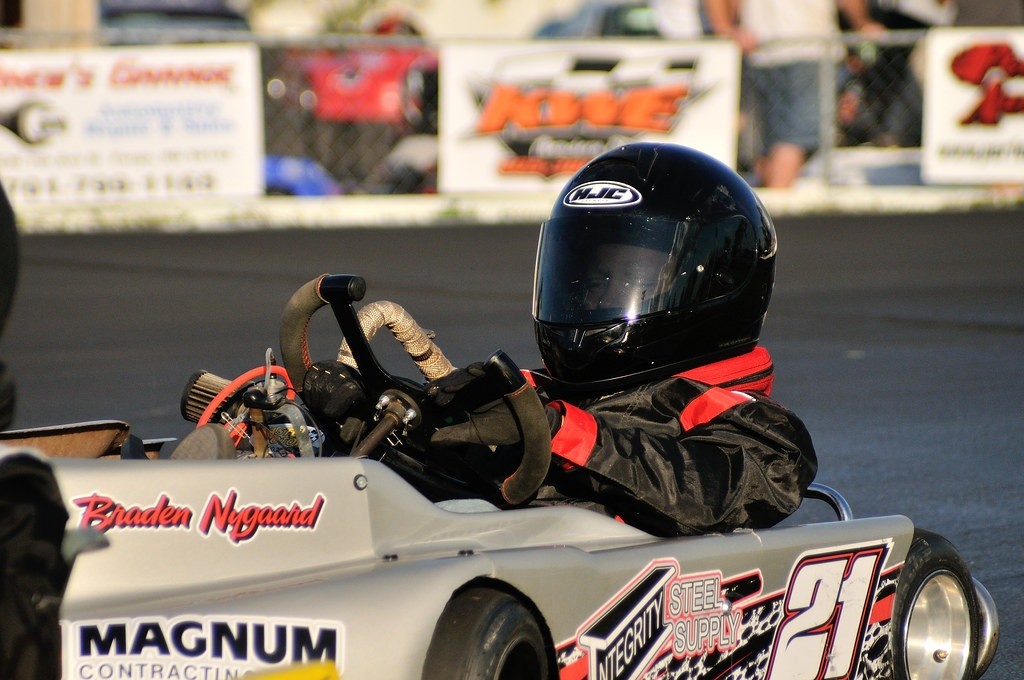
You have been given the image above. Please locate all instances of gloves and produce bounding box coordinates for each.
[302,359,370,422]
[425,361,561,447]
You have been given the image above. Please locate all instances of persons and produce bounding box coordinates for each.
[700,1,887,190]
[167,142,821,537]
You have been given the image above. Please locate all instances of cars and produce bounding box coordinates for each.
[505,1,937,160]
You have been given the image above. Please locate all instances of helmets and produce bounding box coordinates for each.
[532,139,776,397]
